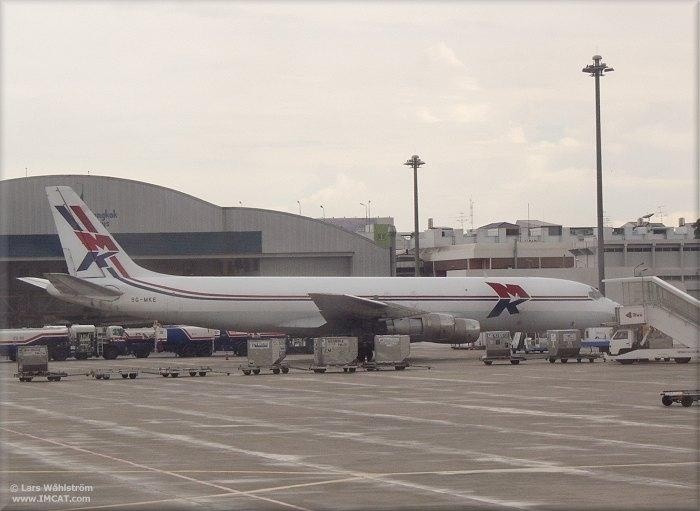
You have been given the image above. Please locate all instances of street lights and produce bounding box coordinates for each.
[580,56,613,295]
[405,154,425,274]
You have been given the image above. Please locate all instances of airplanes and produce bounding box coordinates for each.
[17,185,626,360]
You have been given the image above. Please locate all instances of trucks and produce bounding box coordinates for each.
[524,327,674,356]
[0,322,286,362]
